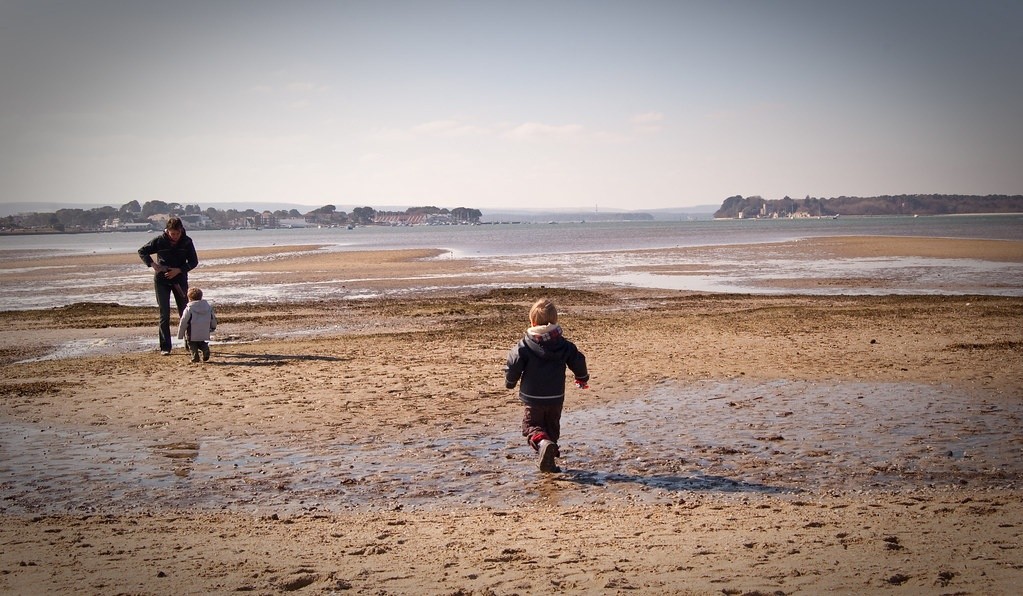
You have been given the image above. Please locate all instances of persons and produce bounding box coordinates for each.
[506,298,590,473]
[178,288,216,362]
[138,217,200,356]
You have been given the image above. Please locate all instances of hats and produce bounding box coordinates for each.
[528,300,558,325]
[187,287,202,300]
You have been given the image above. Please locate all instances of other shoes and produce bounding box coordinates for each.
[161,351,170,355]
[203,352,210,361]
[189,358,199,362]
[538,439,558,472]
